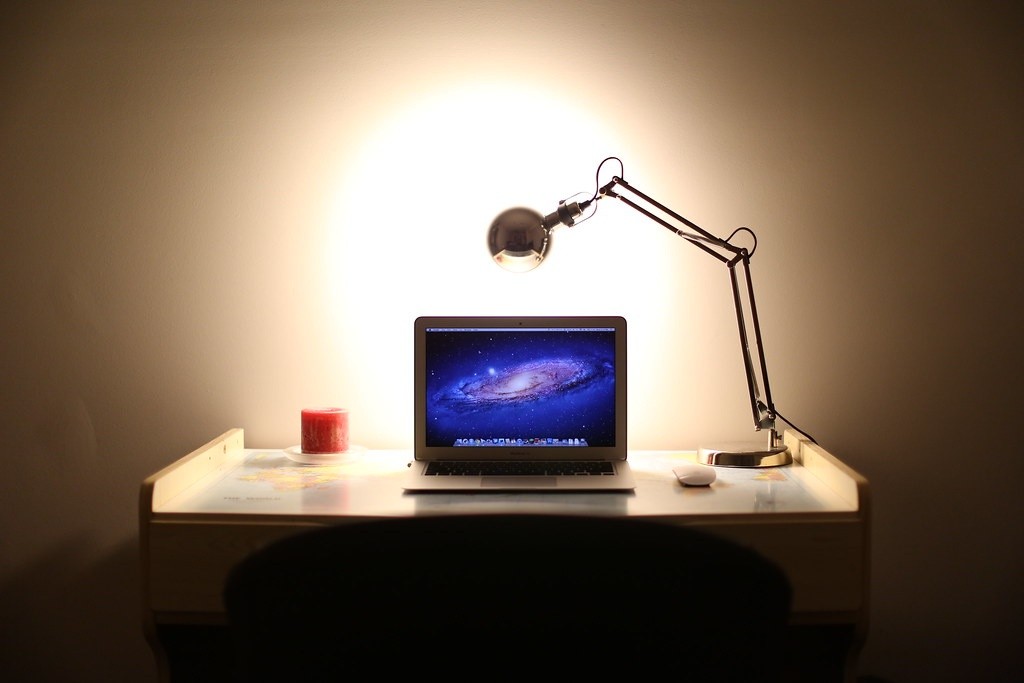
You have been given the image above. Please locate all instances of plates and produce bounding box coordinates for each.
[283,445,364,465]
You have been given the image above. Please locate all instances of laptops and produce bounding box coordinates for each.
[404,316,636,491]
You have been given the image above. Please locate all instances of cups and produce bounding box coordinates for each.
[300,408,350,453]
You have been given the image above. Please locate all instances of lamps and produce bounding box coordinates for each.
[486,156,795,470]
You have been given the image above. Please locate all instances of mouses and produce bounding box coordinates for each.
[672,458,716,485]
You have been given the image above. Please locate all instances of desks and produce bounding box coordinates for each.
[137,427,874,682]
